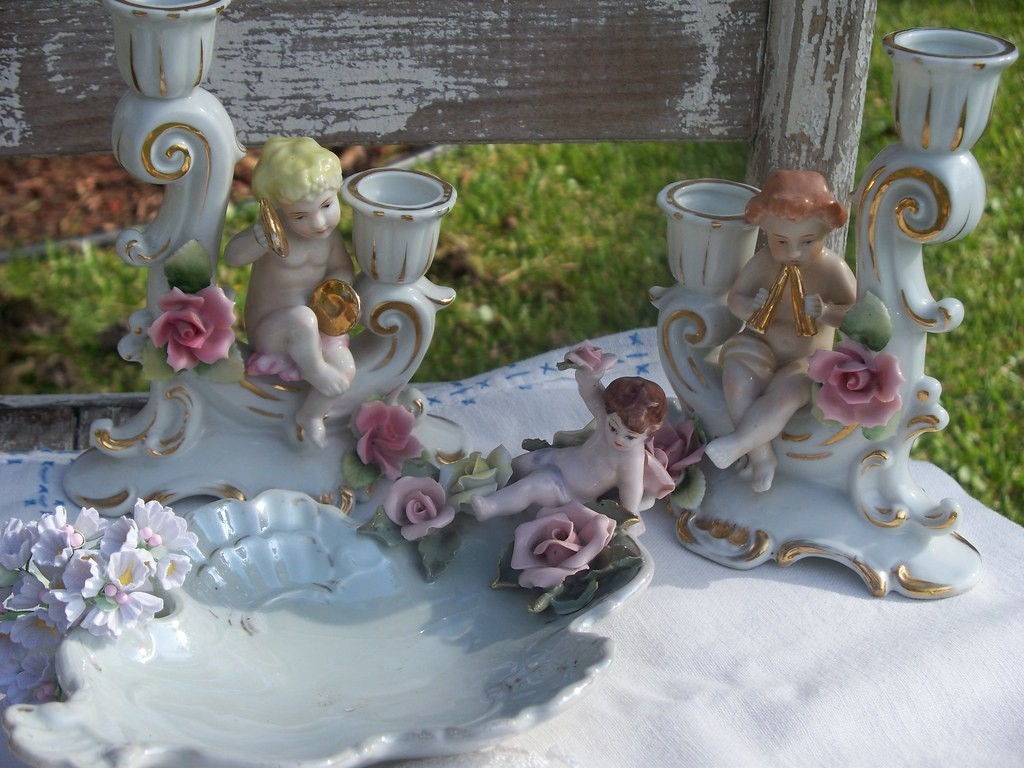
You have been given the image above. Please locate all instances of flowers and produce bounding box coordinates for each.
[364,464,476,580]
[493,498,642,611]
[146,280,240,374]
[336,387,426,489]
[0,498,205,650]
[814,288,901,443]
